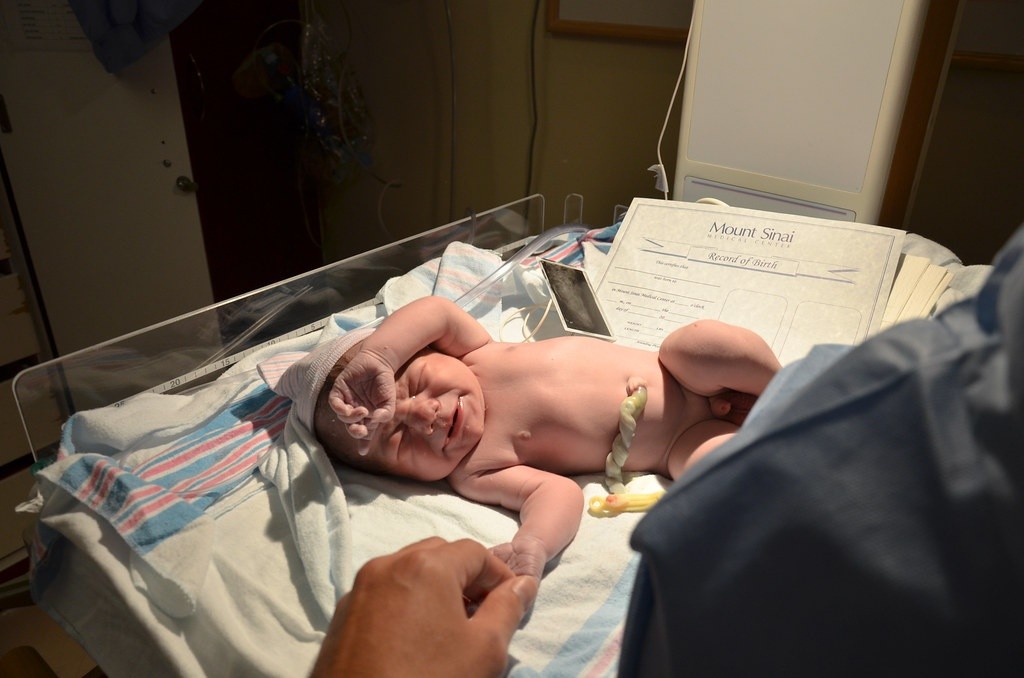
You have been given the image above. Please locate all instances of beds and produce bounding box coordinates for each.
[12,194,991,678]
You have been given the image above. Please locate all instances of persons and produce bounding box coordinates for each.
[256,296,784,588]
[311,223,1024,678]
